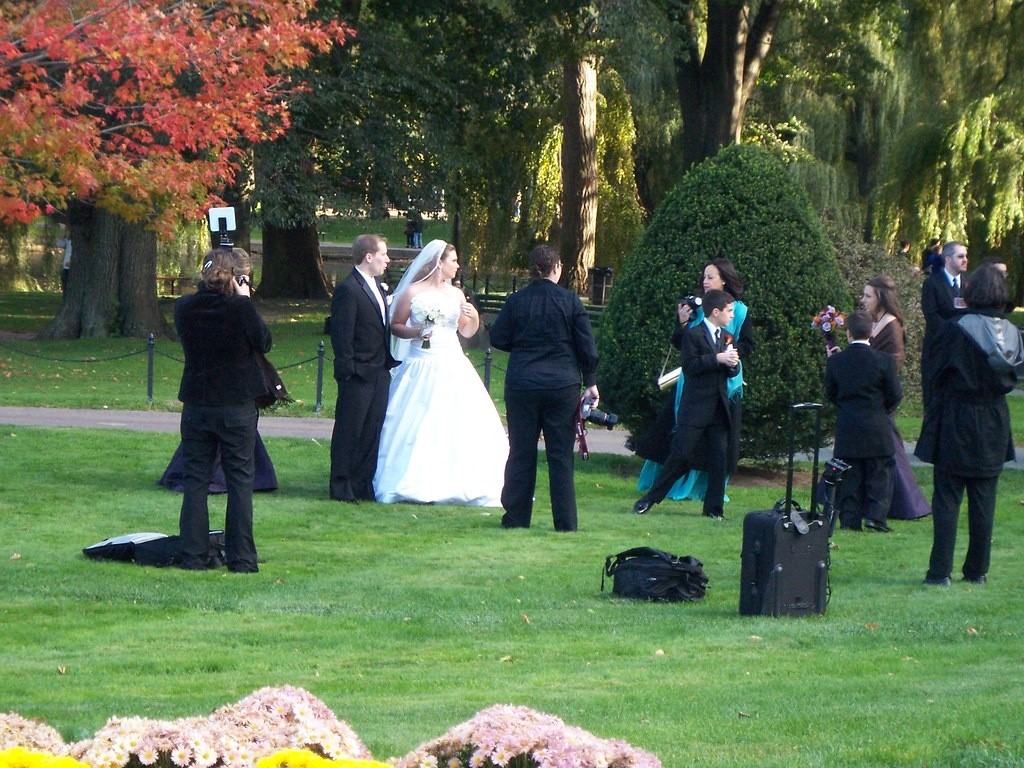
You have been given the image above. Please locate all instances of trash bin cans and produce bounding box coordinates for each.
[588,267,614,305]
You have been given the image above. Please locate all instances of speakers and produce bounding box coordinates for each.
[740,507,829,618]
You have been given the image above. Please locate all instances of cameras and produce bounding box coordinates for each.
[578,398,619,431]
[208,206,243,288]
[681,295,702,321]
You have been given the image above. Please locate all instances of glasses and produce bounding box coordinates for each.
[951,254,968,259]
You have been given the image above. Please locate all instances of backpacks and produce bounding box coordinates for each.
[601,547,707,602]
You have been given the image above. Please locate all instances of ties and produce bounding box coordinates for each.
[953,279,960,299]
[715,329,719,349]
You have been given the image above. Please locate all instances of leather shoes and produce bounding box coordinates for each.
[840,524,863,532]
[922,577,950,586]
[701,511,724,521]
[864,519,892,533]
[962,576,986,584]
[634,496,656,514]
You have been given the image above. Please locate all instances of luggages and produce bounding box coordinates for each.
[740,400,830,618]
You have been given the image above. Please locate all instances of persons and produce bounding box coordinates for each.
[914,266,1024,588]
[330,234,402,504]
[899,237,1008,421]
[826,277,932,531]
[371,239,510,508]
[634,289,742,521]
[156,248,295,575]
[824,310,902,533]
[57,229,73,299]
[488,246,600,533]
[637,257,754,501]
[404,213,423,249]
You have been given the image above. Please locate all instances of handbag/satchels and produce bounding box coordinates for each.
[659,366,682,390]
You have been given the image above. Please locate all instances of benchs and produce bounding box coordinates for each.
[157,276,191,295]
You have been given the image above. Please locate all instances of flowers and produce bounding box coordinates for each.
[409,288,454,349]
[725,333,733,345]
[810,305,846,355]
